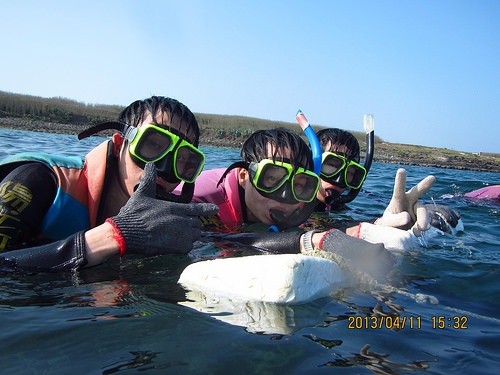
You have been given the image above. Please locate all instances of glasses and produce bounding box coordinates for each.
[217,157,320,205]
[78,121,205,184]
[321,152,366,189]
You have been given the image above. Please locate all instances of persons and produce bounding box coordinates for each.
[1,95,437,299]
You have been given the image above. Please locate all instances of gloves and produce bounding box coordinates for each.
[319,229,392,279]
[358,222,421,251]
[106,161,218,256]
[374,168,436,237]
